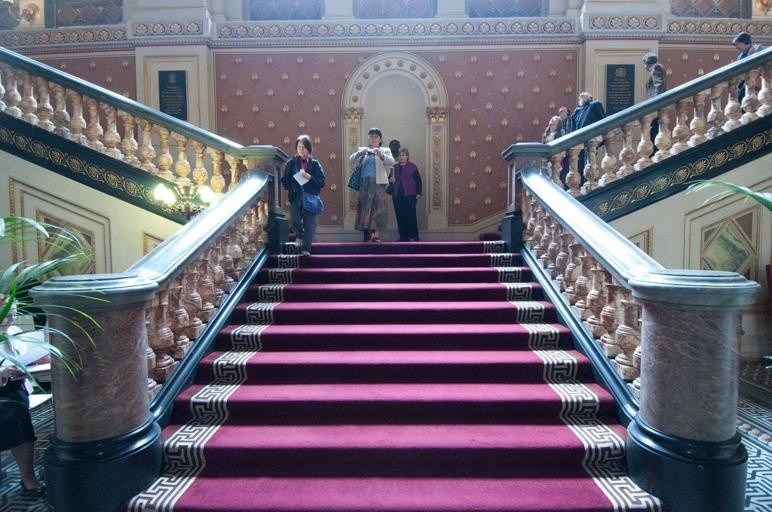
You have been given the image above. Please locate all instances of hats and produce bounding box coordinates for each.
[642,53,659,63]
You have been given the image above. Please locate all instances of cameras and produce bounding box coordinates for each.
[366,150,377,155]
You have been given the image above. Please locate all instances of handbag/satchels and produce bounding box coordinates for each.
[302,192,324,213]
[386,184,392,196]
[347,156,364,190]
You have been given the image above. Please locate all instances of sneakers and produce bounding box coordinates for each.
[294,235,310,257]
[362,230,420,243]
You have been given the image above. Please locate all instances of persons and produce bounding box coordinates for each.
[642,52,667,159]
[388,147,422,242]
[0,296,52,493]
[732,32,766,115]
[388,140,415,243]
[541,92,606,192]
[280,134,326,257]
[349,127,395,243]
